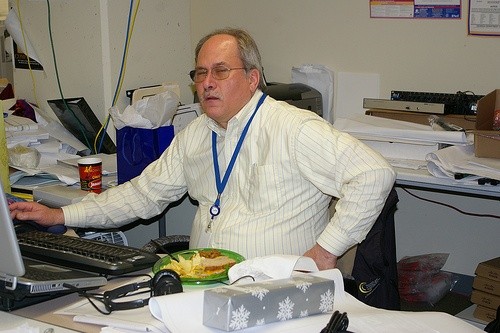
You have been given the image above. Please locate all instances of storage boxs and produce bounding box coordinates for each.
[202,274,334,332]
[470,257,500,322]
[474,89,499,159]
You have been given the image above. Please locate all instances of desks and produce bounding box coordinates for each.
[0,254,183,333]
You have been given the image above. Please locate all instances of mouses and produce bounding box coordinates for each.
[14,223,25,234]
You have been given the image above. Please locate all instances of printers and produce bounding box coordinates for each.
[266,80,324,117]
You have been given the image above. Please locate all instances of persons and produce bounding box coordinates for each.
[8,28,397,271]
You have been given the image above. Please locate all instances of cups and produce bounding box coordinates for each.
[77,159,103,194]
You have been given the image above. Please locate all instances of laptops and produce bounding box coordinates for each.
[0,178,108,294]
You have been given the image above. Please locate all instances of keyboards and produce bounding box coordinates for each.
[14,224,161,276]
[391,90,485,115]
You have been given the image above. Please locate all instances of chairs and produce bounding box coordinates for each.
[143,182,360,276]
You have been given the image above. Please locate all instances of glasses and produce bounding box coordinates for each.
[188,64,256,83]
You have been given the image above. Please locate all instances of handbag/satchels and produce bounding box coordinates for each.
[47,96,117,154]
[116,124,176,186]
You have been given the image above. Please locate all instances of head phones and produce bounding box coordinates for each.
[103,269,183,311]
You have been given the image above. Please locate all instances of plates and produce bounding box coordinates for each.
[153,248,246,285]
